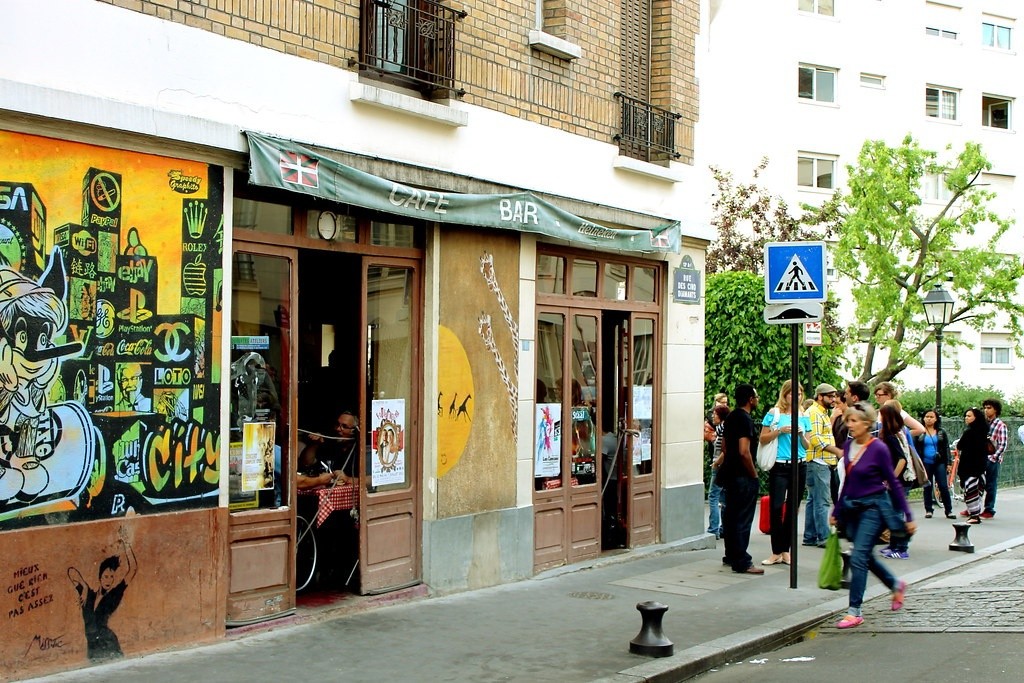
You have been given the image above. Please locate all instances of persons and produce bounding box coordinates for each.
[804,381,925,559]
[274,411,360,583]
[960,399,1007,519]
[957,409,989,524]
[914,410,956,519]
[600,420,617,526]
[537,376,594,482]
[830,404,915,628]
[705,383,764,574]
[759,379,813,565]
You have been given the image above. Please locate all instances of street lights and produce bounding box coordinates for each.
[922,284,955,425]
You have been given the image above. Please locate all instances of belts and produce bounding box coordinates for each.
[774,458,807,464]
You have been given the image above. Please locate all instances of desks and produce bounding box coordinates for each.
[296,476,371,529]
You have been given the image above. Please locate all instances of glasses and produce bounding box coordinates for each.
[852,403,865,412]
[717,400,728,405]
[336,420,356,431]
[874,393,888,397]
[753,395,759,400]
[822,393,837,400]
[969,405,977,410]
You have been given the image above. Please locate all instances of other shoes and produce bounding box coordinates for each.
[715,535,720,540]
[721,538,909,590]
[892,583,906,611]
[837,615,865,629]
[721,532,724,538]
[965,515,982,524]
[946,513,956,519]
[979,511,993,519]
[960,510,968,516]
[925,512,933,518]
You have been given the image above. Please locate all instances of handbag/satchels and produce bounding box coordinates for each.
[934,449,945,462]
[986,438,996,455]
[818,525,843,590]
[714,468,726,487]
[909,446,929,486]
[756,407,780,472]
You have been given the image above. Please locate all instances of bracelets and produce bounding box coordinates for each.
[778,427,782,434]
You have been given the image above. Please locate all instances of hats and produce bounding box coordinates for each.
[816,383,837,393]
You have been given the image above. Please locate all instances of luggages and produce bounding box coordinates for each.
[759,495,786,534]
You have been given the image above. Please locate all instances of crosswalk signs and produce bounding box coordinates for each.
[764,242,828,303]
